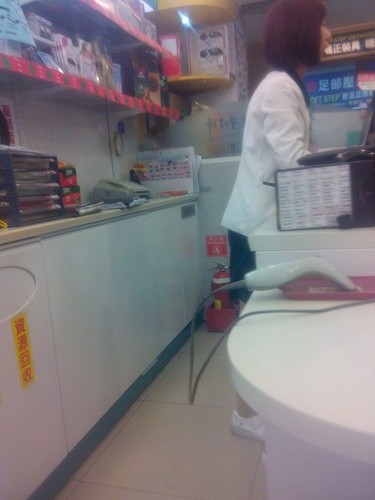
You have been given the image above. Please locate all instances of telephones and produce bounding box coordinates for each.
[93,176,151,203]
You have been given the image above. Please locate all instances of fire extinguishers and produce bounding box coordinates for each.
[208,260,234,309]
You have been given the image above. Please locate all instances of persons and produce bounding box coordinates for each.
[222,0,330,442]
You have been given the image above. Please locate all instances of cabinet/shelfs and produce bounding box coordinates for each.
[0,0,375,500]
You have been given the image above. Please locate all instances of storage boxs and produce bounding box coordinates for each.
[206,301,237,332]
